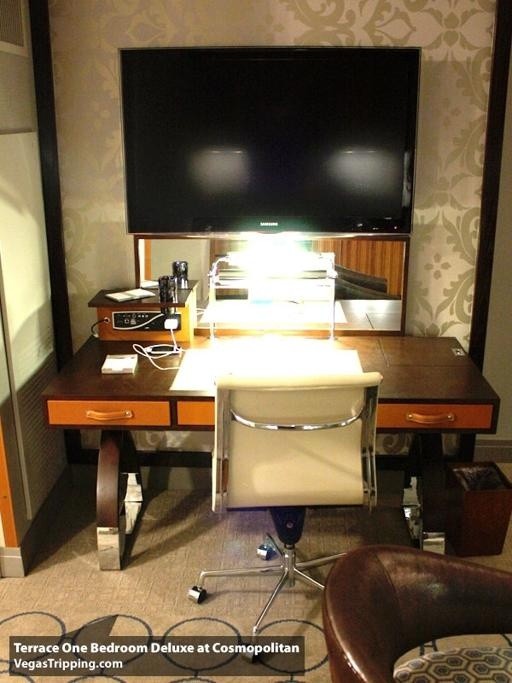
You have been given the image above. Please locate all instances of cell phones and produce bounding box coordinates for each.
[151,345,182,354]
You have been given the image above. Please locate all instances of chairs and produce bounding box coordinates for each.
[322,546,512,681]
[186,372,384,662]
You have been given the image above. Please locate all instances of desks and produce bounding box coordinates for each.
[42,328,500,573]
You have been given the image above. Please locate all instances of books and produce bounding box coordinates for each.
[100,351,138,374]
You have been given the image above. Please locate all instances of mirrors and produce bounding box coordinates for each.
[134,235,410,335]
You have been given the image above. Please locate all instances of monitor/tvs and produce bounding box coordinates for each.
[118,45,422,240]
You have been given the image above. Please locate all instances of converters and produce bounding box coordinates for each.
[165,319,178,329]
